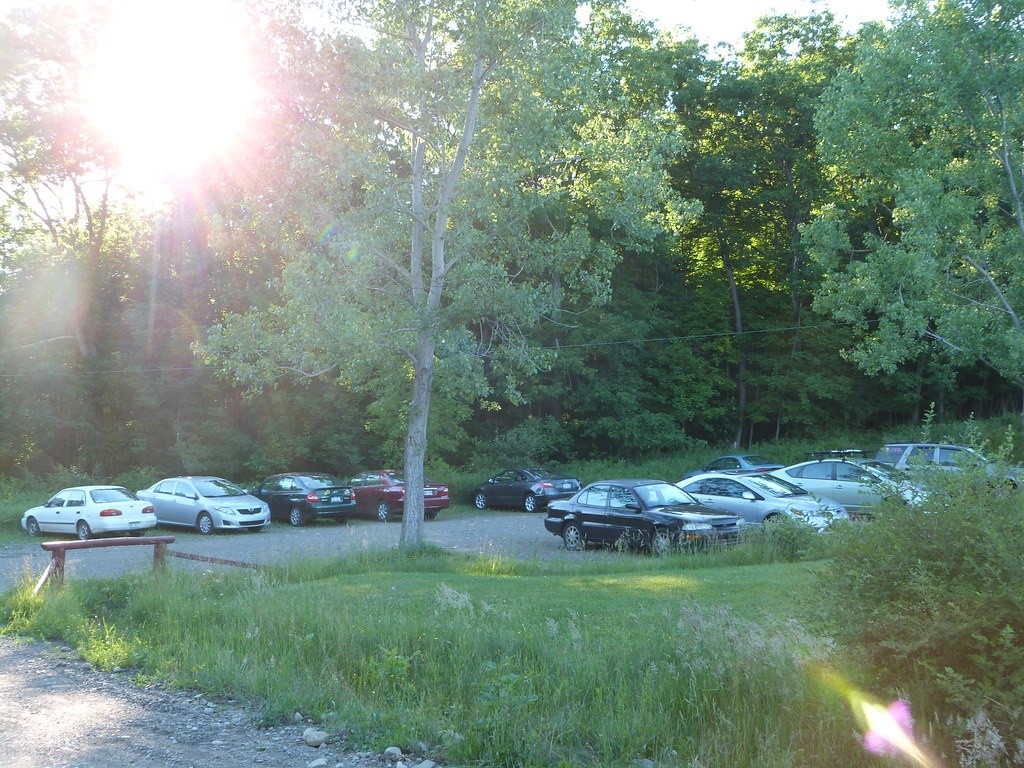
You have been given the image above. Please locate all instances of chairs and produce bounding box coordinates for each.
[638,487,653,505]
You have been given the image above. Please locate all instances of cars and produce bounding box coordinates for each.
[766,449,944,524]
[250,472,358,527]
[874,444,1024,500]
[20,485,157,541]
[543,479,746,559]
[648,472,849,533]
[681,455,786,480]
[471,466,584,513]
[346,469,449,523]
[137,476,271,535]
